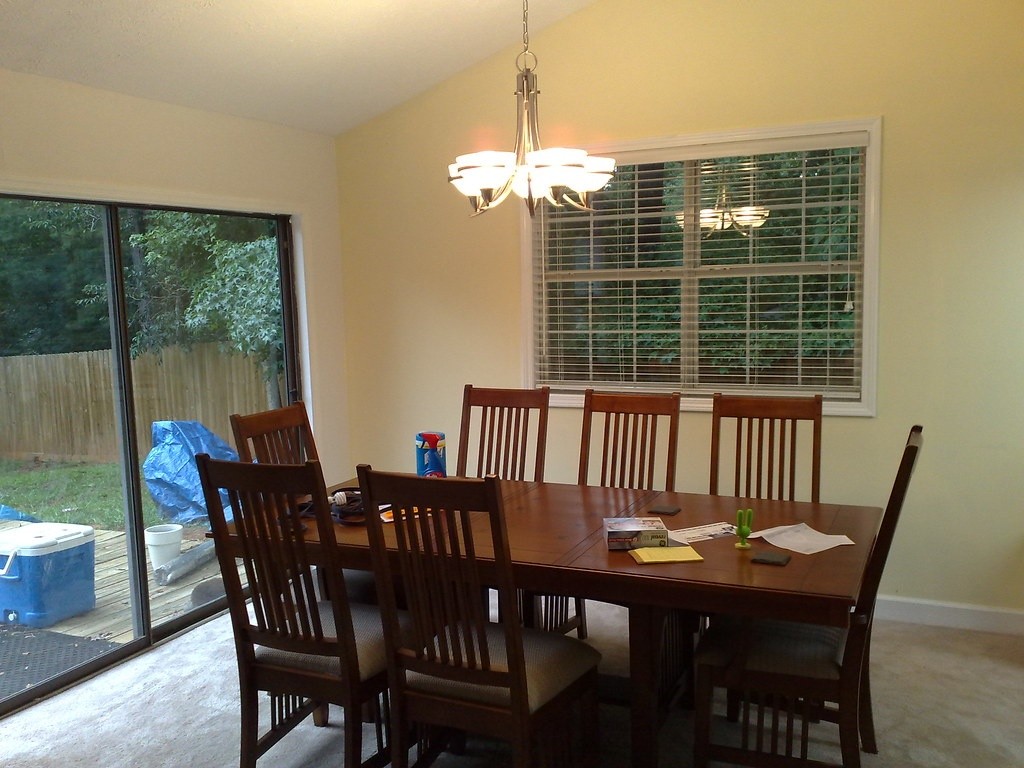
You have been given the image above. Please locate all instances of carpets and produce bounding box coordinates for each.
[0,624,123,699]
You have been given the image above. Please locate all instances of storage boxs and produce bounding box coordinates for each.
[0,522,95,627]
[602,516,668,550]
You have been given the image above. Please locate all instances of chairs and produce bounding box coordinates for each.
[195,382,922,768]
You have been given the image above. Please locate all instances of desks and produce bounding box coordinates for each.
[204,478,884,768]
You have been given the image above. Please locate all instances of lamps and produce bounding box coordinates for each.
[446,1,616,217]
[675,159,769,237]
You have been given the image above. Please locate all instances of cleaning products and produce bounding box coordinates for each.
[417,432,448,479]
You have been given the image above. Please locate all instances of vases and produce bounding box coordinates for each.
[144,523,183,569]
